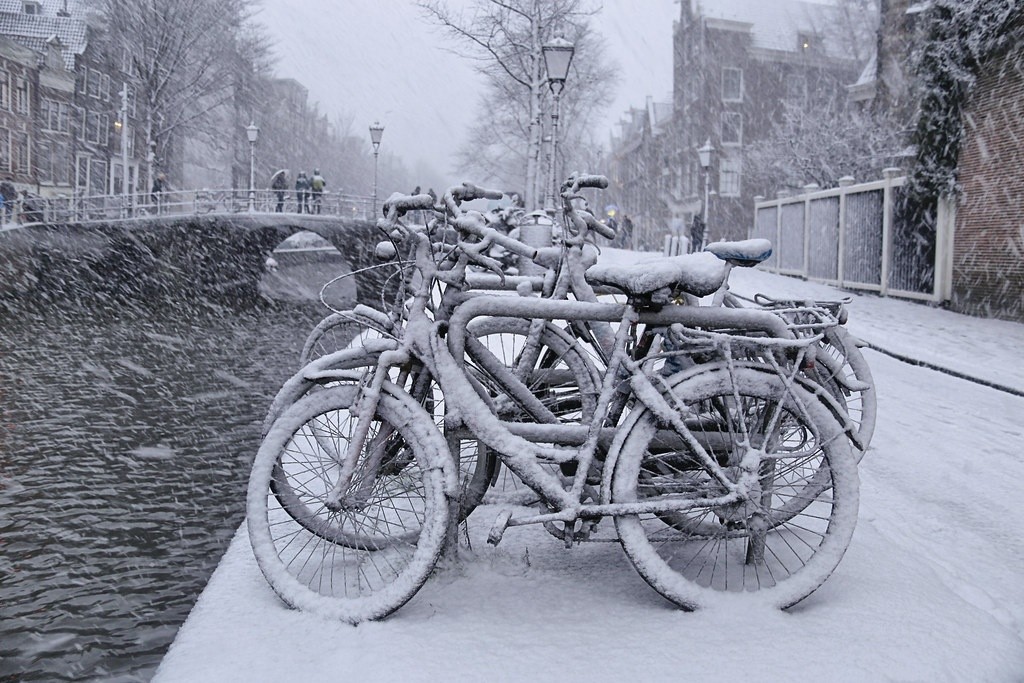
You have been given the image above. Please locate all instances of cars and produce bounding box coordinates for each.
[1,193,65,224]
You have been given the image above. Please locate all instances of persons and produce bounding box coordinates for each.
[296,171,311,214]
[427,188,437,226]
[606,218,618,248]
[271,171,289,212]
[151,173,165,207]
[690,214,706,255]
[308,168,326,214]
[617,215,633,251]
[411,186,421,225]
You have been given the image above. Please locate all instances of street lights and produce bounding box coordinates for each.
[604,203,618,219]
[244,120,261,211]
[369,121,385,219]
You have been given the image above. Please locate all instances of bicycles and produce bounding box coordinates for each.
[193,184,241,214]
[259,187,298,214]
[311,188,359,218]
[246,169,877,629]
[145,191,174,214]
[45,189,99,222]
